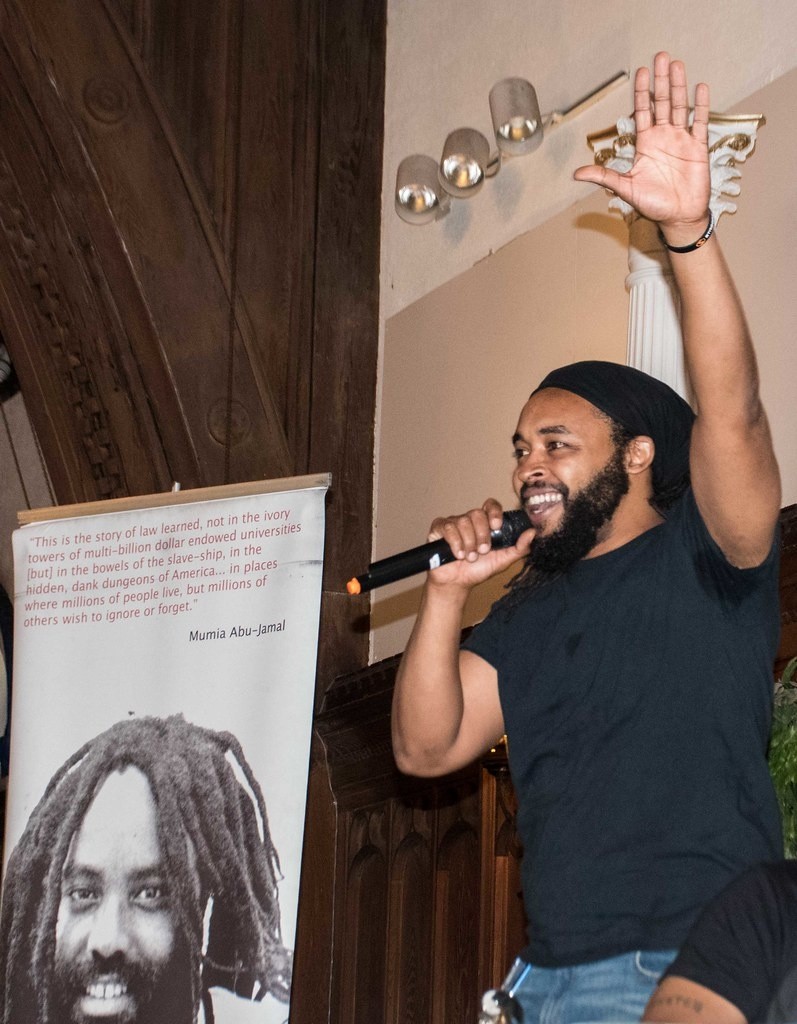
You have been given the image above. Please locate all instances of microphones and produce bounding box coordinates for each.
[347,508,533,595]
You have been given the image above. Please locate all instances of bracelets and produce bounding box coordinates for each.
[658,207,715,253]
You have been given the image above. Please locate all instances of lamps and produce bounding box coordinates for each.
[394,157,445,225]
[437,128,489,200]
[489,79,546,158]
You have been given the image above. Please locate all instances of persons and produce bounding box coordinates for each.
[0,714,292,1024]
[389,51,783,1024]
[640,860,797,1024]
